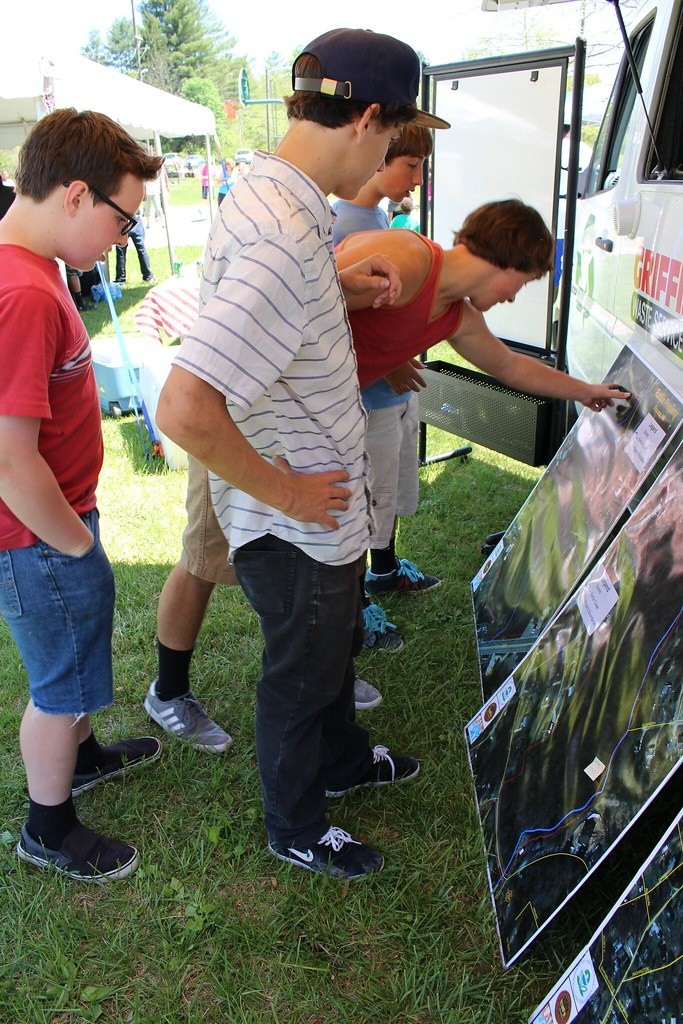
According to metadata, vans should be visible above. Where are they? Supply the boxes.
[563,0,683,421]
[234,148,253,165]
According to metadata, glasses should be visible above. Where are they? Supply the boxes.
[63,178,139,237]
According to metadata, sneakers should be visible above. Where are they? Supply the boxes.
[346,671,383,711]
[323,745,420,799]
[142,678,233,755]
[358,590,405,654]
[363,557,442,597]
[268,822,386,882]
[15,820,141,885]
[66,736,163,799]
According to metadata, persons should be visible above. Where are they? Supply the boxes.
[142,190,634,754]
[387,193,413,223]
[65,263,97,311]
[198,156,250,207]
[389,196,420,234]
[0,169,17,221]
[0,108,166,880]
[141,150,170,229]
[112,209,157,283]
[321,123,443,654]
[152,21,452,882]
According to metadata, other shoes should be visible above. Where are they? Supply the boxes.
[76,303,98,312]
[111,279,126,285]
[141,271,154,284]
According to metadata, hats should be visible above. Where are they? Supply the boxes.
[289,27,451,130]
[399,197,414,215]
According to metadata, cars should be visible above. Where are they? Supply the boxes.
[162,152,207,179]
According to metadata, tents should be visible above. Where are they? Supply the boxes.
[2,35,217,289]
